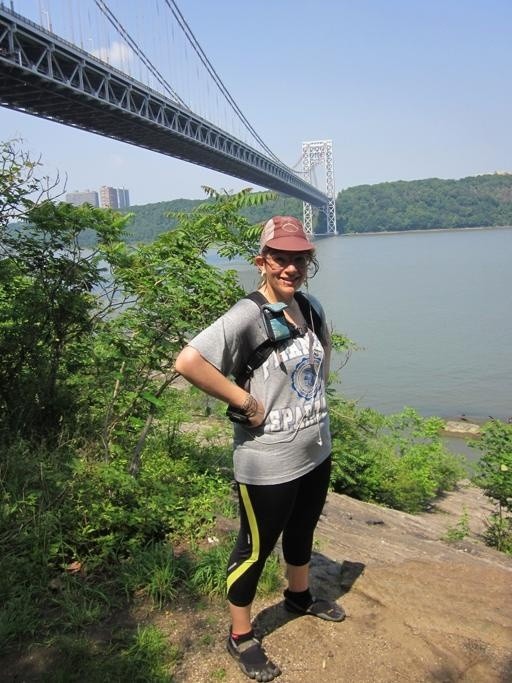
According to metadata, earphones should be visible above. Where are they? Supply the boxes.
[316,437,325,448]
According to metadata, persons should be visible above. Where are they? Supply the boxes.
[172,214,347,683]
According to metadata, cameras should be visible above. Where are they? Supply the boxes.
[225,404,248,427]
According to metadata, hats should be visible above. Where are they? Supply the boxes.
[258,216,316,254]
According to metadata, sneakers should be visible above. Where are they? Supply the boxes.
[284,592,345,622]
[227,635,280,682]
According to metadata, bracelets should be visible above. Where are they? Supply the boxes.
[240,393,258,418]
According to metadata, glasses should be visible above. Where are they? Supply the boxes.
[262,254,312,270]
